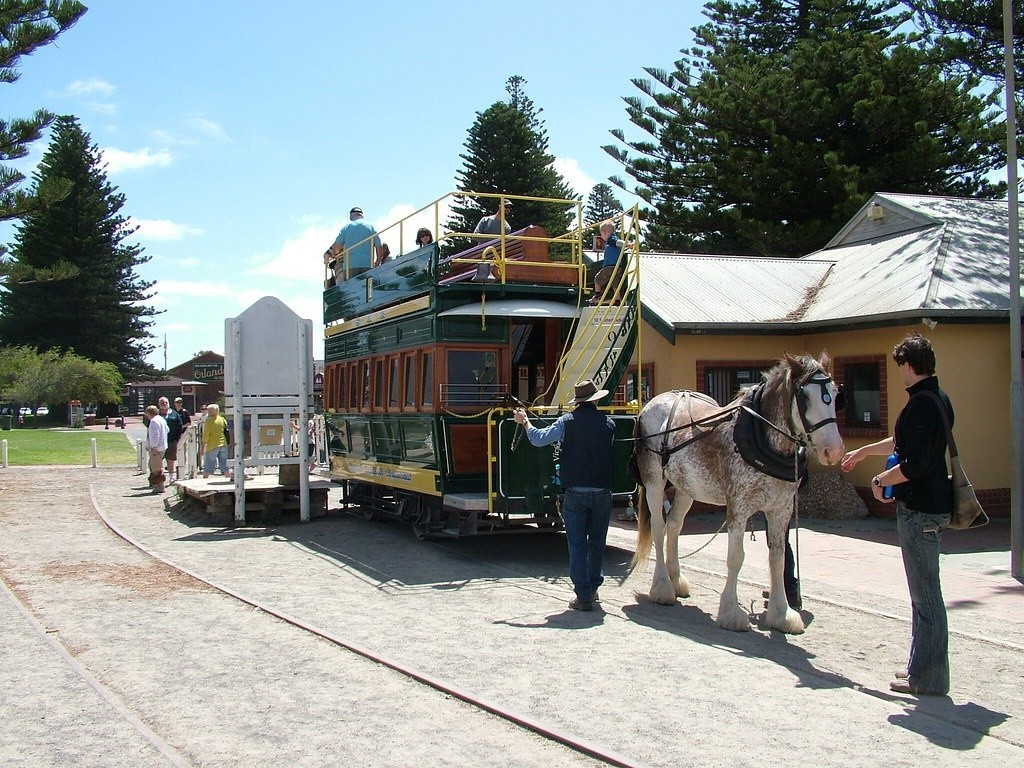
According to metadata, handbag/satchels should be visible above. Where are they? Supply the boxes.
[223,426,231,446]
[947,433,990,531]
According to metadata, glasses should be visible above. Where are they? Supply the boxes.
[202,408,206,410]
[420,233,431,239]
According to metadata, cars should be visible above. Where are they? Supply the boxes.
[20,408,32,415]
[2,408,13,415]
[37,407,48,415]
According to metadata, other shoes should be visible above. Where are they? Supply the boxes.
[586,292,604,305]
[890,667,922,695]
[570,588,600,610]
[146,482,163,495]
[612,300,621,306]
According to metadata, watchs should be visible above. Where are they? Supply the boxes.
[874,475,882,487]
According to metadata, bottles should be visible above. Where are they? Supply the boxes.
[881,448,899,498]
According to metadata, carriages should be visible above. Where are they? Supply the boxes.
[324,191,846,634]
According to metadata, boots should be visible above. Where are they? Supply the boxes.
[762,575,803,609]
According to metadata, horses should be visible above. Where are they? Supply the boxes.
[633,347,845,633]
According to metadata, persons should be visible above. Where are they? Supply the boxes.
[586,221,639,305]
[290,419,317,471]
[324,206,394,284]
[145,396,191,494]
[841,335,955,696]
[514,379,616,610]
[472,198,513,245]
[415,227,433,248]
[197,403,253,481]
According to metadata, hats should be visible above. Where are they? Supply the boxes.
[349,208,364,215]
[567,380,611,406]
[499,200,513,207]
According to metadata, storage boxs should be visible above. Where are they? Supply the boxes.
[279,464,301,486]
[260,425,282,444]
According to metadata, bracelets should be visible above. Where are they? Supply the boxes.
[525,421,528,425]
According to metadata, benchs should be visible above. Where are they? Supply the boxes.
[438,226,579,285]
